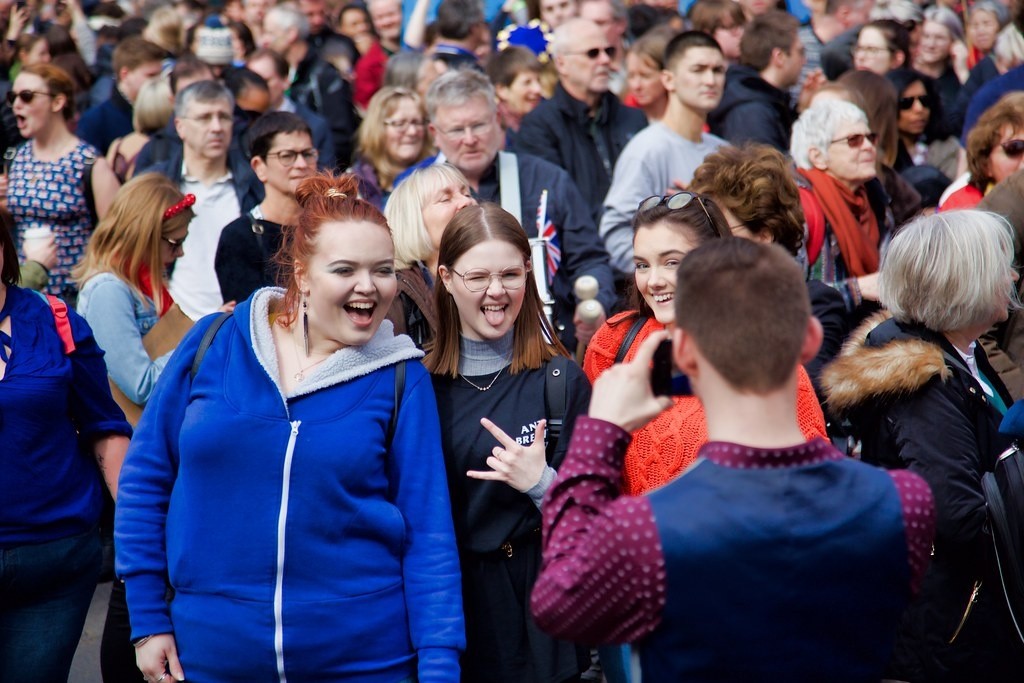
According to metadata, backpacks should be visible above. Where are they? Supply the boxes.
[982,441,1024,643]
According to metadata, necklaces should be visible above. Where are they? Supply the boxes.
[458,352,512,391]
[292,332,325,380]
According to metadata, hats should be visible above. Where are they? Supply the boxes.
[191,15,234,65]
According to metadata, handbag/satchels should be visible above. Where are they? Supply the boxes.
[100,578,174,683]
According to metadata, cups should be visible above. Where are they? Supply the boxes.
[23,227,52,254]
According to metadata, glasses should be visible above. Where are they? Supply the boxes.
[438,122,494,139]
[895,94,933,109]
[850,45,895,56]
[566,46,615,58]
[385,119,430,131]
[1000,139,1024,156]
[161,233,184,253]
[451,268,532,292]
[830,133,879,147]
[6,90,55,108]
[639,192,719,237]
[264,147,319,165]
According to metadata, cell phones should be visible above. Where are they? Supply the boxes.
[649,338,695,396]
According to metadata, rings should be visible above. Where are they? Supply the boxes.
[144,676,148,680]
[497,450,504,458]
[158,673,166,681]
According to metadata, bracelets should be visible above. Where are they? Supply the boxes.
[133,635,153,648]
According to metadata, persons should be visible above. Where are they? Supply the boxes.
[0,0,1024,683]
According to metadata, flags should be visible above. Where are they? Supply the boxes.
[535,187,561,287]
